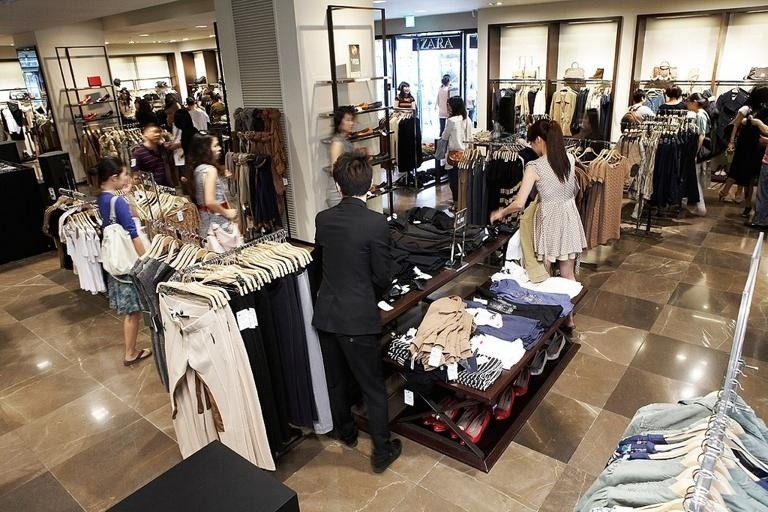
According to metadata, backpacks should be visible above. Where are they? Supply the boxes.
[621,106,641,132]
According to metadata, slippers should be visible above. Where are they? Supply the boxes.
[124,350,152,366]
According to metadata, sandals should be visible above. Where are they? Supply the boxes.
[720,192,743,203]
[449,203,458,212]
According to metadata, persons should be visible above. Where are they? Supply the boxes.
[490,119,588,330]
[394,82,416,108]
[97,156,153,366]
[312,153,401,474]
[441,95,474,211]
[326,106,367,209]
[118,86,227,185]
[628,88,655,130]
[718,86,768,228]
[684,92,712,215]
[437,73,477,133]
[183,133,243,252]
[655,85,688,116]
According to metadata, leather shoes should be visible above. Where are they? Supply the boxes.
[369,152,388,160]
[367,182,390,198]
[688,207,707,216]
[350,127,379,139]
[357,101,381,109]
[743,207,767,229]
[424,398,493,445]
[495,367,531,419]
[346,438,402,472]
[79,94,113,120]
[530,333,566,375]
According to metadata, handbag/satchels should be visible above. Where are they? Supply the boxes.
[102,196,152,275]
[207,223,244,254]
[747,67,768,79]
[653,62,676,79]
[697,113,722,164]
[565,63,584,77]
[448,151,463,166]
[512,57,538,80]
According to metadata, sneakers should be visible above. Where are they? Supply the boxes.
[711,165,727,182]
